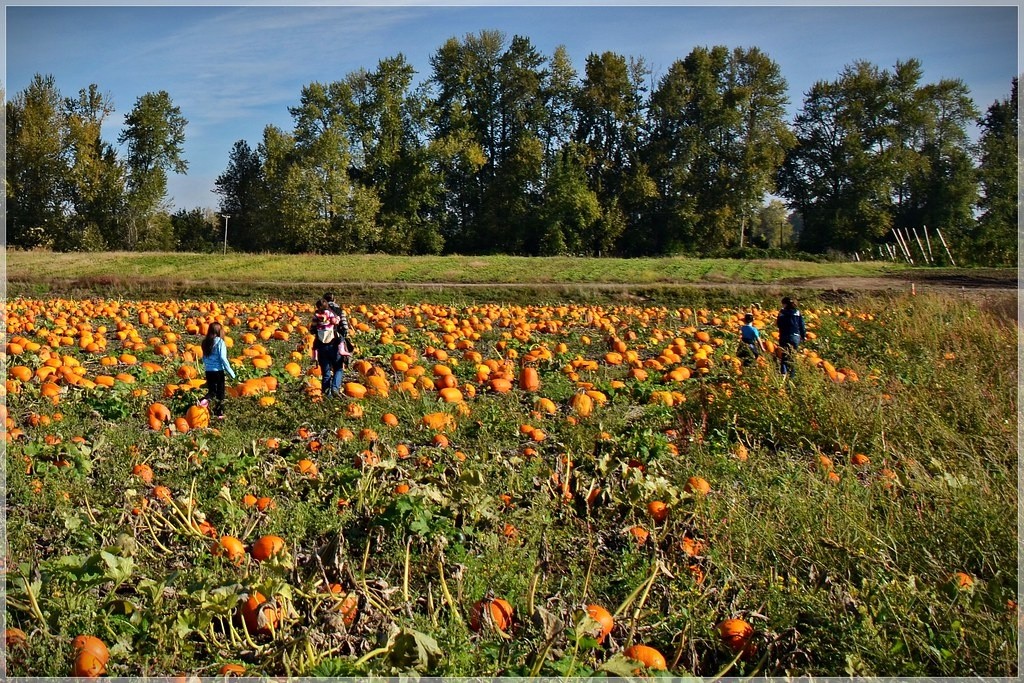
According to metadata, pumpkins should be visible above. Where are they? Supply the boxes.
[0,298,973,682]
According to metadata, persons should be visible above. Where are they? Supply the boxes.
[199,321,236,419]
[309,291,354,400]
[776,297,807,378]
[739,313,766,367]
